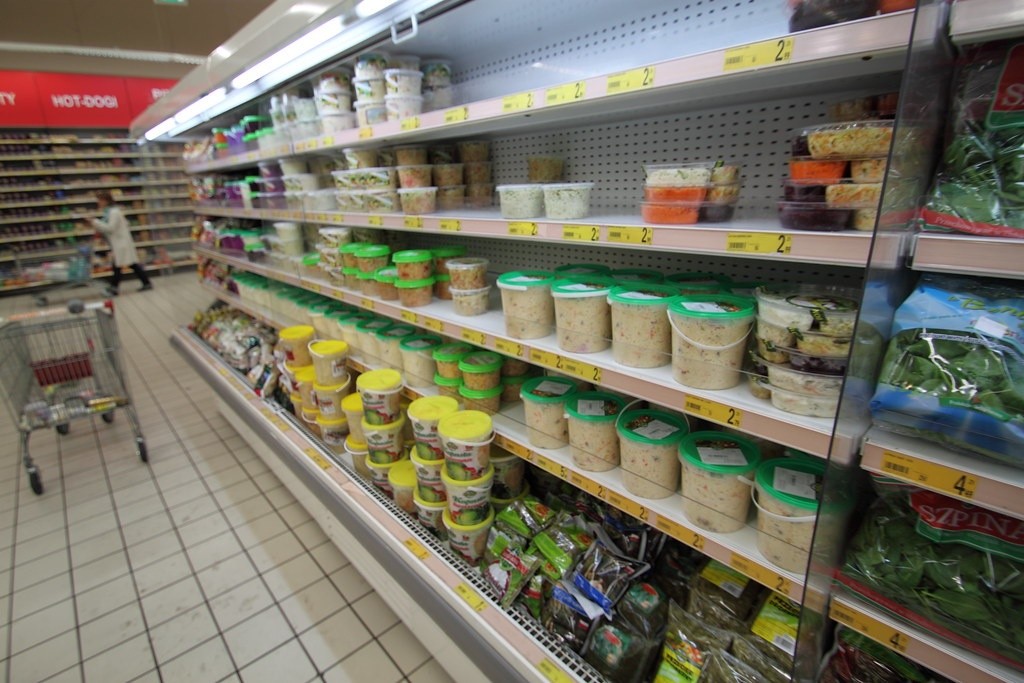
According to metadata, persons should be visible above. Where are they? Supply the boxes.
[84,193,153,296]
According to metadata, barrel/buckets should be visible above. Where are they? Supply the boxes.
[209,49,850,575]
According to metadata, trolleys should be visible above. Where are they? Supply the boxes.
[1,300,149,496]
[10,231,109,306]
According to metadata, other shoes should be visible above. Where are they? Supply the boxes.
[136,283,152,293]
[104,285,120,296]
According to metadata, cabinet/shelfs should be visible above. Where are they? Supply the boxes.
[0,138,198,293]
[182,0,1024,683]
